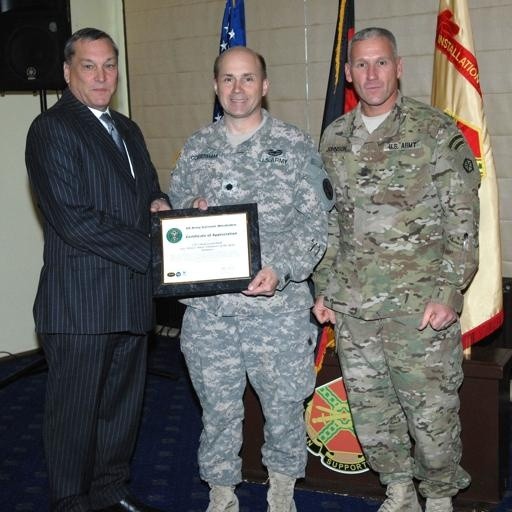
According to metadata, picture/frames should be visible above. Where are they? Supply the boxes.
[151,203,262,299]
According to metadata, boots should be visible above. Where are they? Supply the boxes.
[265,476,299,511]
[425,496,453,512]
[377,478,423,512]
[205,482,239,512]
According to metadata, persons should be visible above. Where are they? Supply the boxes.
[23,27,177,511]
[308,25,483,511]
[166,44,340,512]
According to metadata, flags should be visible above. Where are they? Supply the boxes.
[211,1,248,123]
[300,1,357,380]
[428,1,509,361]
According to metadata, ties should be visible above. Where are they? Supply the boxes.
[98,112,131,173]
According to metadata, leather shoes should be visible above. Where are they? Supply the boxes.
[99,494,161,512]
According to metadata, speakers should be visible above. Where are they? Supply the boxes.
[0,0,72,91]
